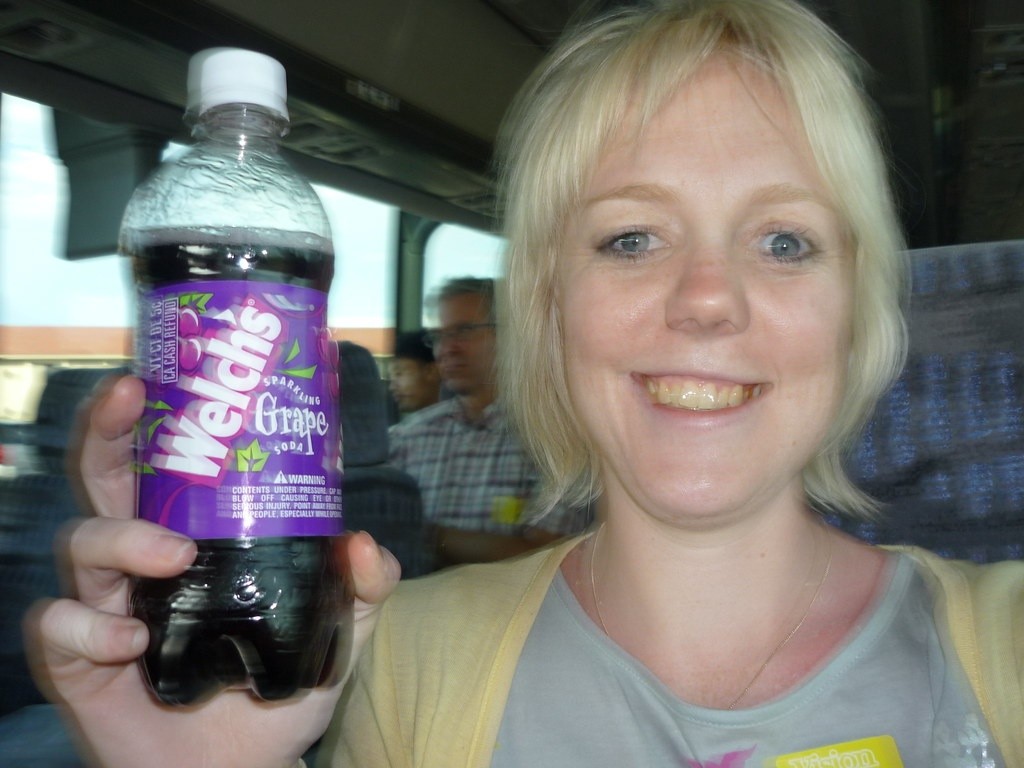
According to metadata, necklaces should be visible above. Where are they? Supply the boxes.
[589,518,836,711]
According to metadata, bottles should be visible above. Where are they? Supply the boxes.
[115,46,346,711]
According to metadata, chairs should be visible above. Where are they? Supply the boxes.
[0,240,1024,768]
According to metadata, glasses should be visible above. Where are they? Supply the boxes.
[422,323,495,348]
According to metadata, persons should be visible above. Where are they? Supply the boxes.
[20,0,1024,768]
[387,276,589,563]
[388,327,442,415]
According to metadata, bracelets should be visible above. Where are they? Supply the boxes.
[428,525,447,556]
